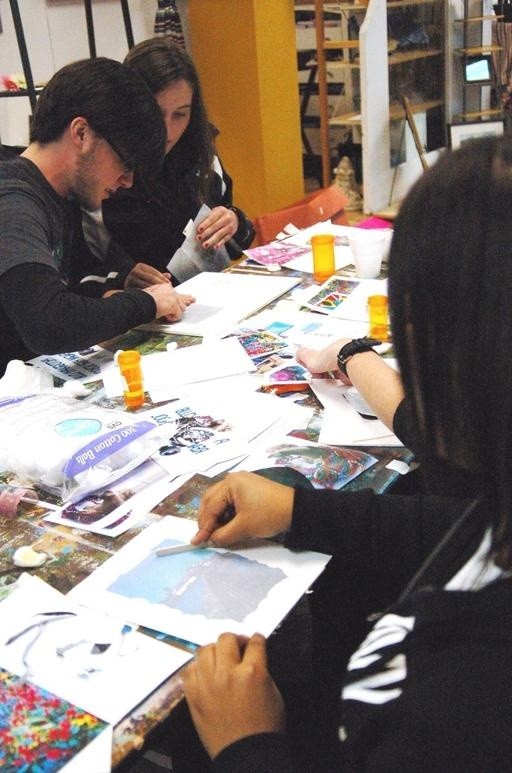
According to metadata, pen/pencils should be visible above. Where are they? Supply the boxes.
[19,497,64,512]
[155,541,206,557]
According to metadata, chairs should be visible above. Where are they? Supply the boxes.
[255,187,348,247]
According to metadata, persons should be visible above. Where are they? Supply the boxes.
[1,58,185,378]
[297,339,429,456]
[184,132,512,773]
[100,38,255,285]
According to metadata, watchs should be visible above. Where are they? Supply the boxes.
[338,336,382,377]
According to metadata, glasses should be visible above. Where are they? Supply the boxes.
[102,133,138,172]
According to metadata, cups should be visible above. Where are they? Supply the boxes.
[116,350,145,412]
[311,233,337,281]
[367,296,390,344]
[349,230,386,278]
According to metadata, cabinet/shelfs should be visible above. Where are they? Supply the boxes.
[312,1,504,194]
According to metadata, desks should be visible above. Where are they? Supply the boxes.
[1,213,412,773]
